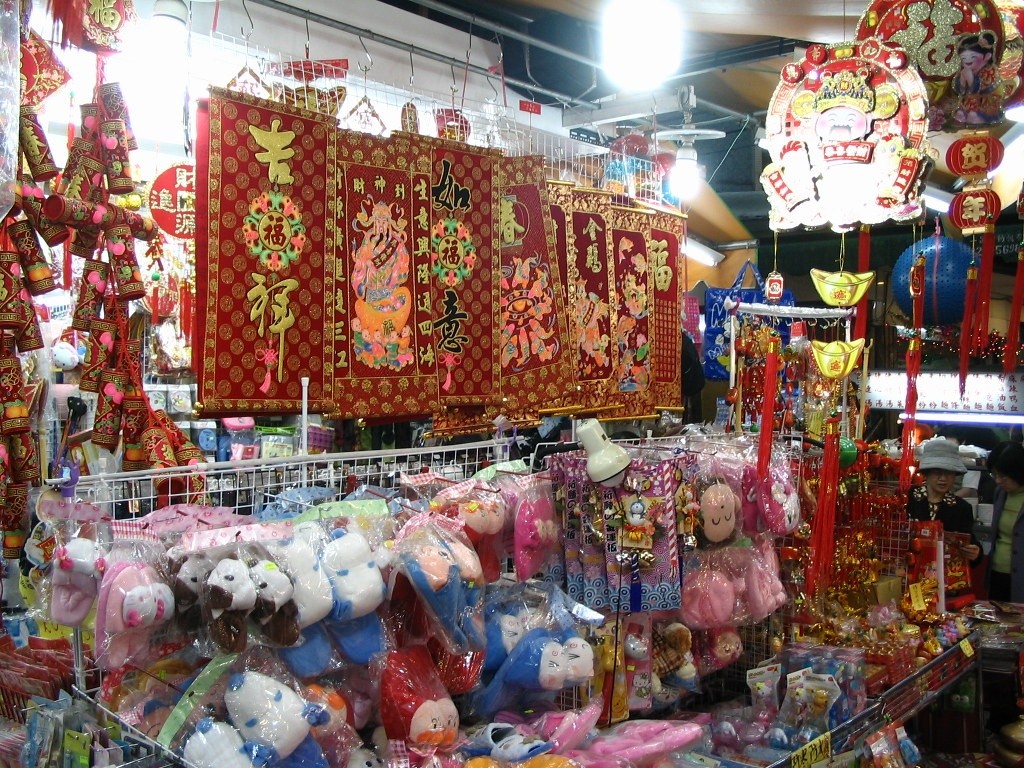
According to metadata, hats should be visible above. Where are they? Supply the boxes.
[918,440,968,475]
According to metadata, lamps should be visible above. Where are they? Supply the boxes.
[534,420,633,483]
[152,0,188,36]
[651,110,727,198]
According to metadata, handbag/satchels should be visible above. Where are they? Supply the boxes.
[703,260,792,383]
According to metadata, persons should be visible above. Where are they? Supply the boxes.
[979,441,1024,604]
[905,439,984,601]
[947,432,981,500]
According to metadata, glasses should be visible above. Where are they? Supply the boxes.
[990,470,1009,481]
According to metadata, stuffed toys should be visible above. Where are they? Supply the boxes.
[650,456,801,704]
[53,477,702,768]
